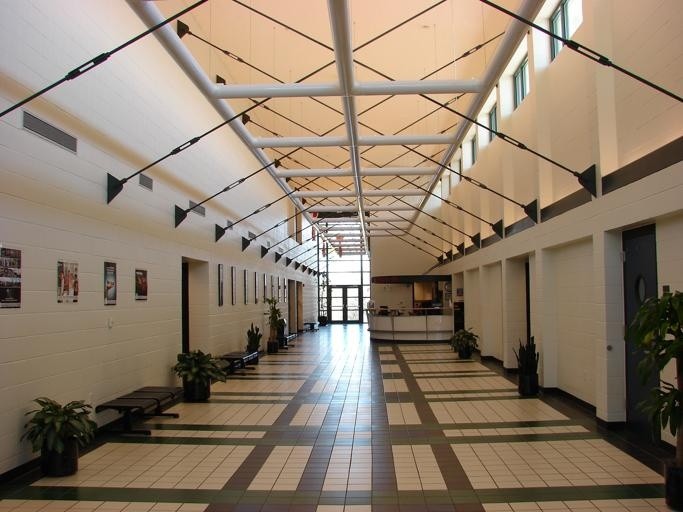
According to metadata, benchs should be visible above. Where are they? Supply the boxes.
[304,323,319,332]
[222,352,258,375]
[96,386,181,435]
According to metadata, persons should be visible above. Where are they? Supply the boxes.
[57,268,78,302]
[141,274,147,296]
[0,248,20,302]
[136,274,142,295]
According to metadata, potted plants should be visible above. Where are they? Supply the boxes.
[172,350,230,401]
[448,328,480,359]
[20,397,98,477]
[512,336,539,397]
[246,322,262,365]
[264,297,286,353]
[624,290,683,512]
